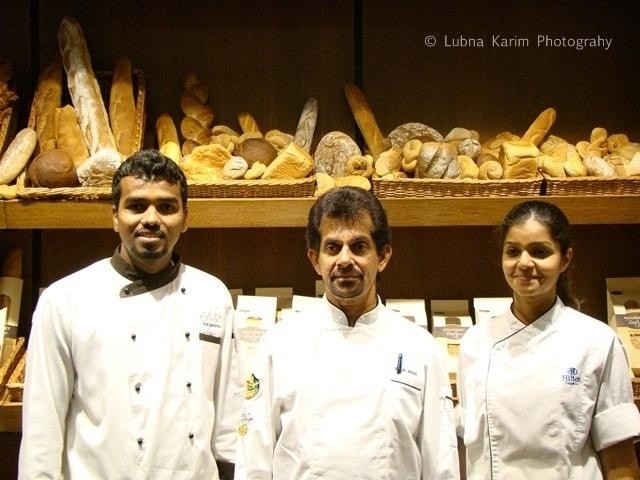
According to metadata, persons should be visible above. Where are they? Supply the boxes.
[17,148,244,480]
[457,201,640,479]
[234,182,462,479]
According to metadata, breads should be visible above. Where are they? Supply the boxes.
[1,249,28,406]
[1,18,639,198]
